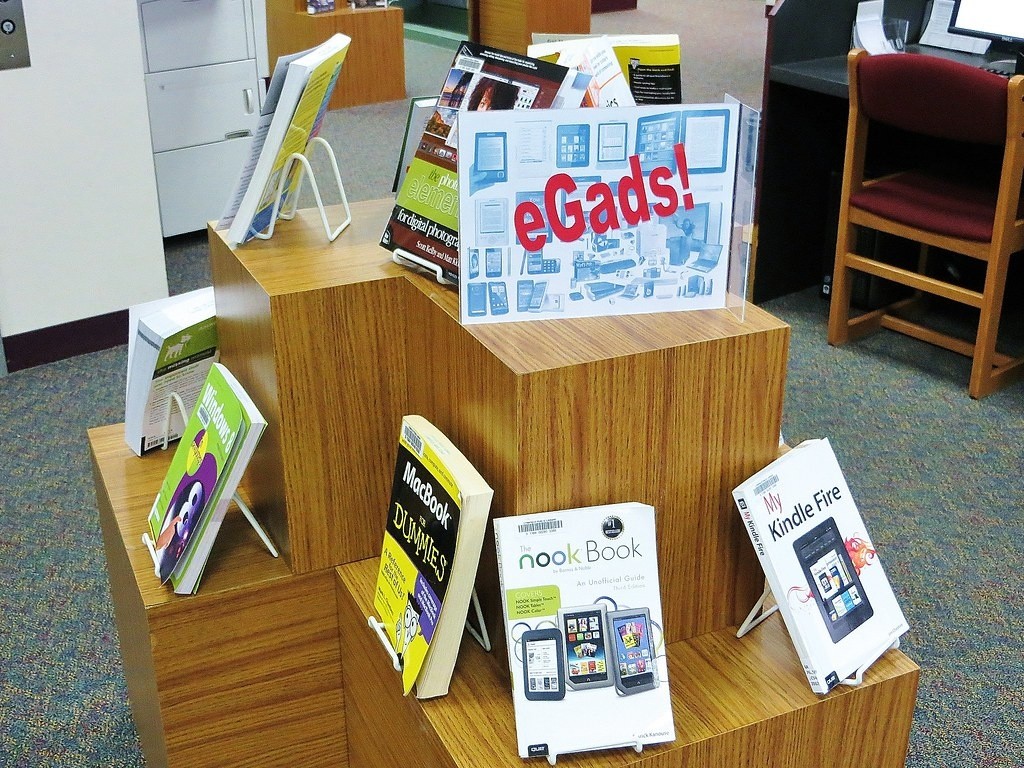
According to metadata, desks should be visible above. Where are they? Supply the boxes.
[748,0,1023,333]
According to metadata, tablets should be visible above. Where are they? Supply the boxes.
[521,604,660,700]
[792,518,875,644]
[468,109,730,316]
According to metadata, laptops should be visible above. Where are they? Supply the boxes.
[687,246,723,274]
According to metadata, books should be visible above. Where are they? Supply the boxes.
[734,437,910,695]
[124,285,217,457]
[493,501,676,758]
[215,33,351,244]
[147,363,268,595]
[373,414,494,699]
[379,33,682,287]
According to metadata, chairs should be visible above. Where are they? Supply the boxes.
[828,48,1024,399]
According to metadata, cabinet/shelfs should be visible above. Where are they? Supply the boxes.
[137,0,270,240]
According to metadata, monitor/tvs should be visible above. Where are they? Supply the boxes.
[947,0,1024,54]
